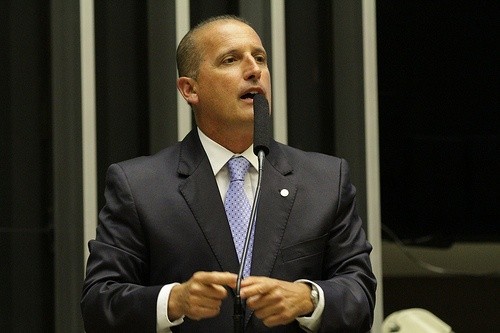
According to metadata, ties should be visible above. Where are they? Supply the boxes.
[223,158,256,279]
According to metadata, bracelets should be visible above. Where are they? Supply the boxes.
[299,283,321,320]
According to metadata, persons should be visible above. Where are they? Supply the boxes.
[84,14,377,332]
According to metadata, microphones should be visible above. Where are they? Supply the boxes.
[253,94,270,154]
